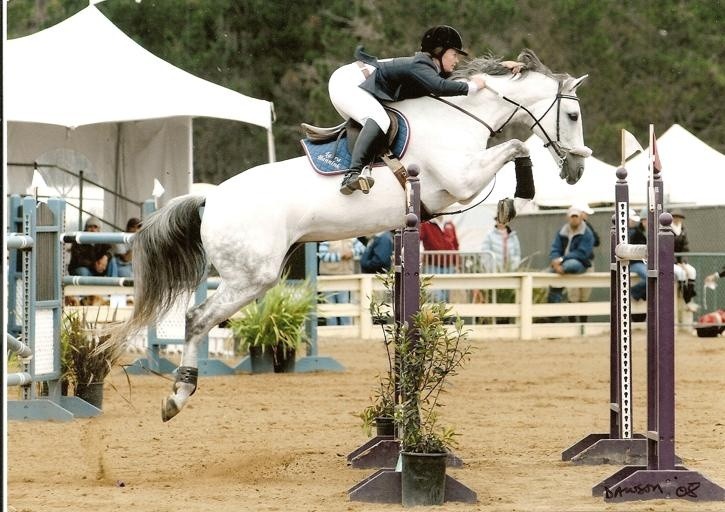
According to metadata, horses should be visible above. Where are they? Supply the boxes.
[76,47,589,423]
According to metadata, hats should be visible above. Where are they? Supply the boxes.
[85,216,101,228]
[128,217,143,228]
[567,203,596,219]
[626,208,689,222]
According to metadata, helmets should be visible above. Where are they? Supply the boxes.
[420,26,469,58]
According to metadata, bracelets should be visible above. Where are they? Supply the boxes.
[558,257,564,263]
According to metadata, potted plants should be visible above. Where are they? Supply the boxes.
[224,263,341,376]
[14,306,174,410]
[348,252,477,506]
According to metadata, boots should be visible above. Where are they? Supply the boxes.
[683,279,700,313]
[340,120,388,195]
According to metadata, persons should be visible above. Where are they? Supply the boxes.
[619,205,725,316]
[113,217,144,278]
[315,237,367,325]
[327,24,524,196]
[417,214,461,323]
[360,229,393,325]
[479,218,521,274]
[67,216,118,278]
[567,203,602,322]
[547,206,596,322]
